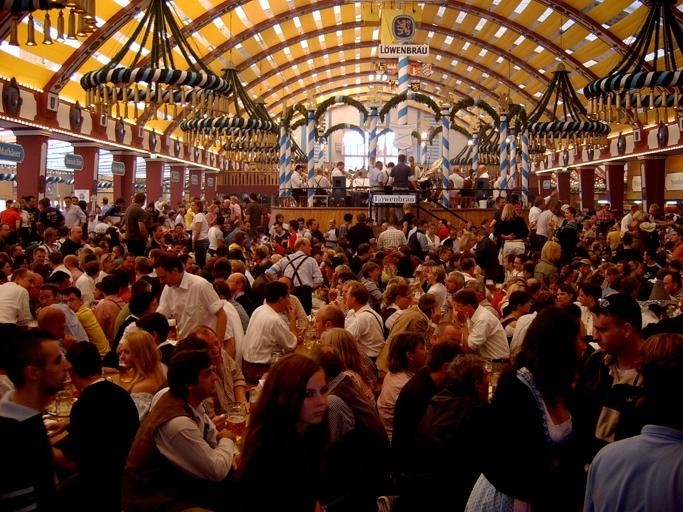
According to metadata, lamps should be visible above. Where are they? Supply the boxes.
[447,109,546,172]
[79,1,229,120]
[0,1,98,48]
[583,2,682,129]
[516,65,611,154]
[418,157,450,185]
[180,64,277,148]
[216,98,306,175]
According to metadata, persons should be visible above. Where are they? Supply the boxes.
[289,151,489,208]
[1,183,683,512]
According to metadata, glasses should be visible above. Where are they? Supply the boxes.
[597,297,614,312]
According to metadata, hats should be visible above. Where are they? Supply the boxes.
[578,258,591,265]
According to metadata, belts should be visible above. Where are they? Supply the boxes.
[492,358,511,363]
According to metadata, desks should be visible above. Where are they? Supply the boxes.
[27,264,456,469]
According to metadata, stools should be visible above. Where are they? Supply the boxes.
[274,188,493,211]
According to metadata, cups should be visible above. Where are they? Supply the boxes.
[483,359,501,399]
[61,374,73,390]
[166,318,177,338]
[118,356,134,384]
[296,314,322,354]
[486,279,494,298]
[54,390,72,424]
[224,399,247,443]
